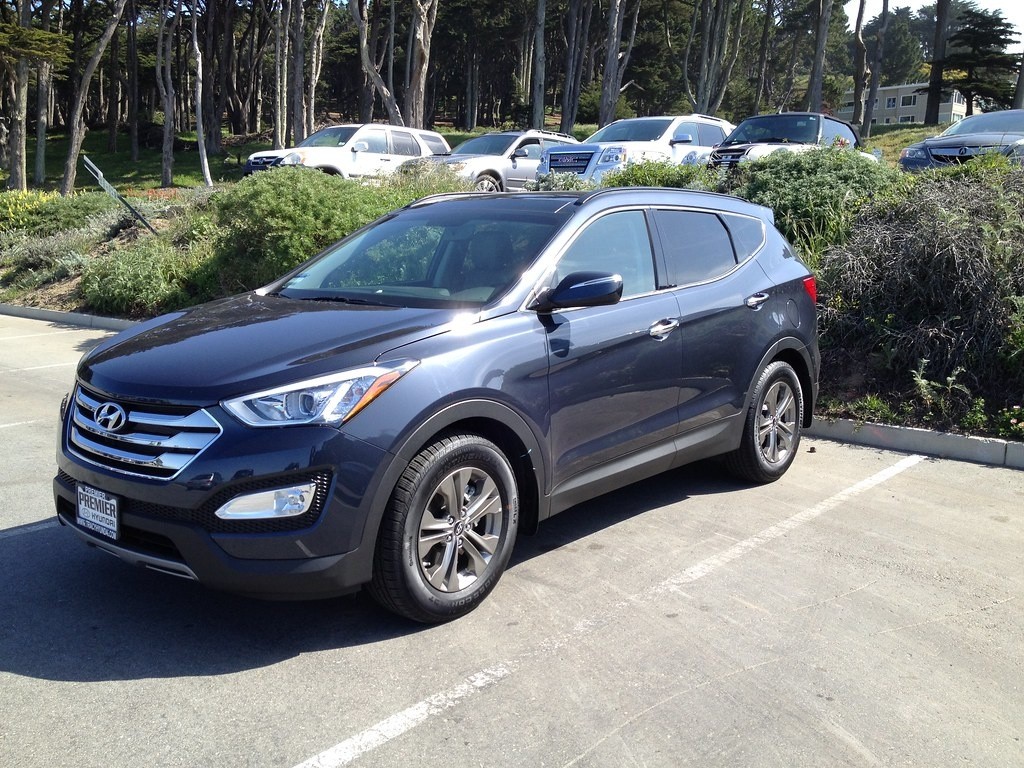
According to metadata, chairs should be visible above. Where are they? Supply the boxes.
[462,231,513,289]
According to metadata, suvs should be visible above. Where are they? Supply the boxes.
[389,127,582,190]
[242,123,453,187]
[706,108,879,182]
[534,112,747,191]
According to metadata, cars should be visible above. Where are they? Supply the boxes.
[50,185,822,626]
[895,108,1024,176]
